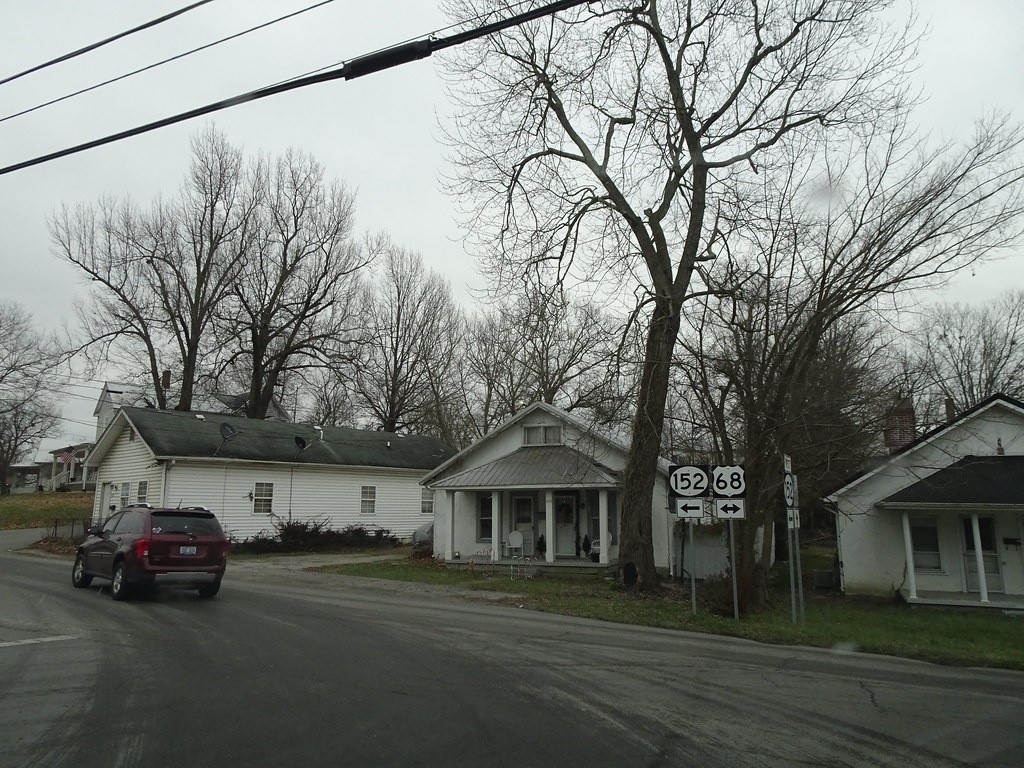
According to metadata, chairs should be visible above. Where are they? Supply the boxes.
[505,531,523,559]
[592,532,613,553]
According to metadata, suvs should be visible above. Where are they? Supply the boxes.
[72,503,231,602]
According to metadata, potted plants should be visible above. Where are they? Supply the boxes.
[582,534,591,559]
[536,533,546,558]
